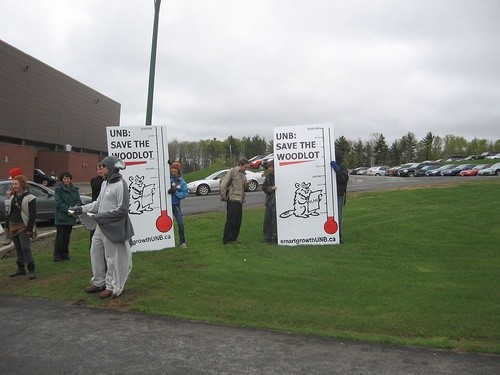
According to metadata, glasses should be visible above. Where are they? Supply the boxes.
[102,163,105,167]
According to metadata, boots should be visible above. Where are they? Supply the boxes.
[27,262,36,279]
[9,261,26,277]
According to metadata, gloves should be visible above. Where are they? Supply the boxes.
[330,161,341,172]
[68,206,83,216]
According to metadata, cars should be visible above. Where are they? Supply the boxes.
[186,167,266,196]
[349,150,500,177]
[248,152,274,169]
[0,179,92,225]
[34,167,58,186]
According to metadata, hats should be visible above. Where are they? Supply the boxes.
[171,162,181,170]
[9,168,22,178]
[101,155,126,181]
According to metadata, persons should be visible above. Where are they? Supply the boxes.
[69,154,135,298]
[0,150,348,280]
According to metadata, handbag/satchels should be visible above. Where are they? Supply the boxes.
[21,211,38,239]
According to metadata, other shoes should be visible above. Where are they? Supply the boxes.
[85,286,104,291]
[99,289,113,298]
[180,243,187,248]
